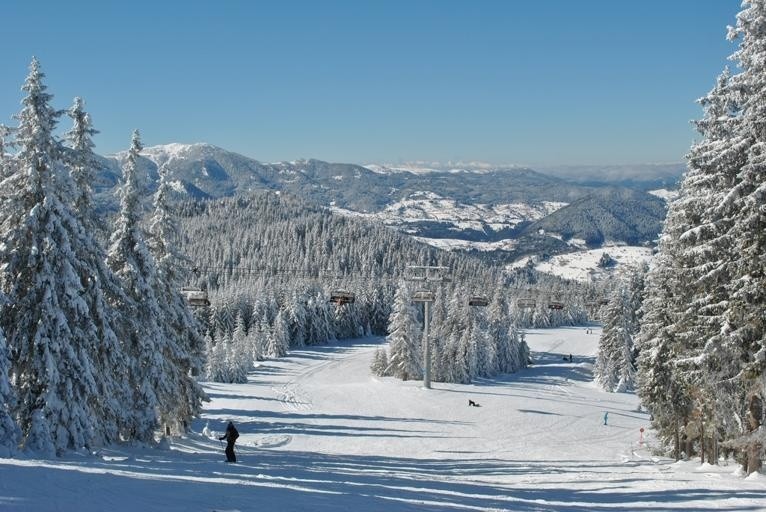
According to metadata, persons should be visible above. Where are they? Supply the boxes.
[605,411,608,426]
[219,421,239,462]
[570,354,572,362]
[469,399,480,407]
